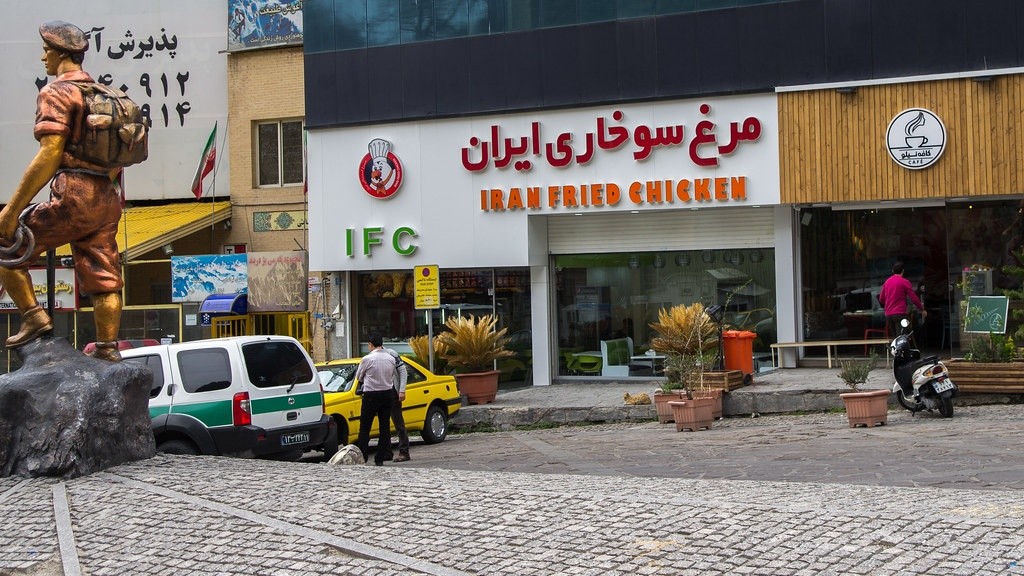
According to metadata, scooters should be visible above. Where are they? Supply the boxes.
[885,318,959,418]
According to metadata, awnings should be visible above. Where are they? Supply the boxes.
[37,200,232,263]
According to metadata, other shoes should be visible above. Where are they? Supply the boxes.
[393,453,411,461]
[375,459,383,466]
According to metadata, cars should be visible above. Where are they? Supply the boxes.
[313,355,461,446]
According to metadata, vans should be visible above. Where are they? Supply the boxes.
[81,335,335,461]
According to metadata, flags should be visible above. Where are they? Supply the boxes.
[192,121,218,199]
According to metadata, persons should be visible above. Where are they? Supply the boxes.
[879,262,927,349]
[0,21,124,364]
[356,332,410,466]
